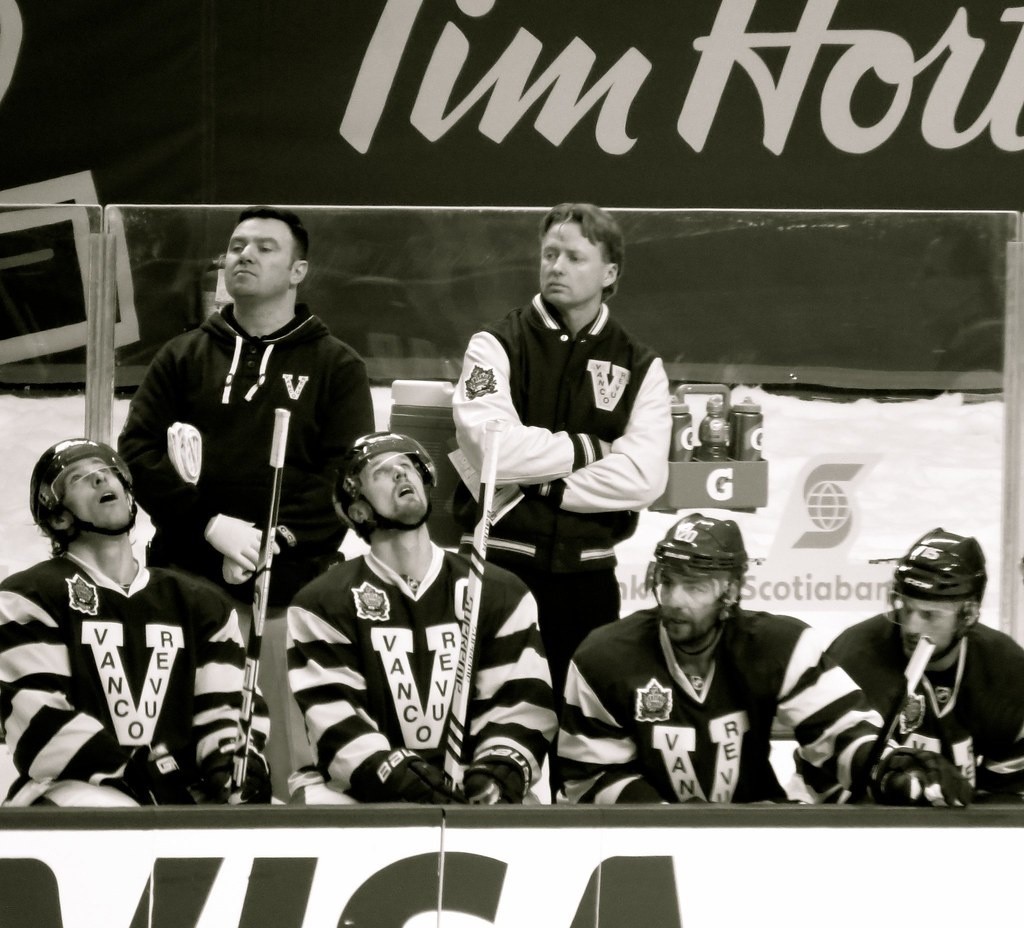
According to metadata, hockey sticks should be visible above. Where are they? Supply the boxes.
[849,640,936,803]
[226,407,294,807]
[442,422,504,797]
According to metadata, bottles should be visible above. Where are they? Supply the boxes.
[669,396,694,461]
[699,397,731,462]
[730,397,765,462]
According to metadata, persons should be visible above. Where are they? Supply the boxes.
[792,524,1024,807]
[556,512,982,809]
[449,199,675,706]
[280,430,554,805]
[114,204,378,811]
[0,439,271,807]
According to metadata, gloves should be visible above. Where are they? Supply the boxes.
[123,743,196,804]
[214,735,272,804]
[365,747,454,804]
[204,514,280,572]
[871,746,971,808]
[461,746,532,805]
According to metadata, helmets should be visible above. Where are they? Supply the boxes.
[892,527,988,601]
[654,512,748,571]
[331,431,437,540]
[30,437,133,545]
